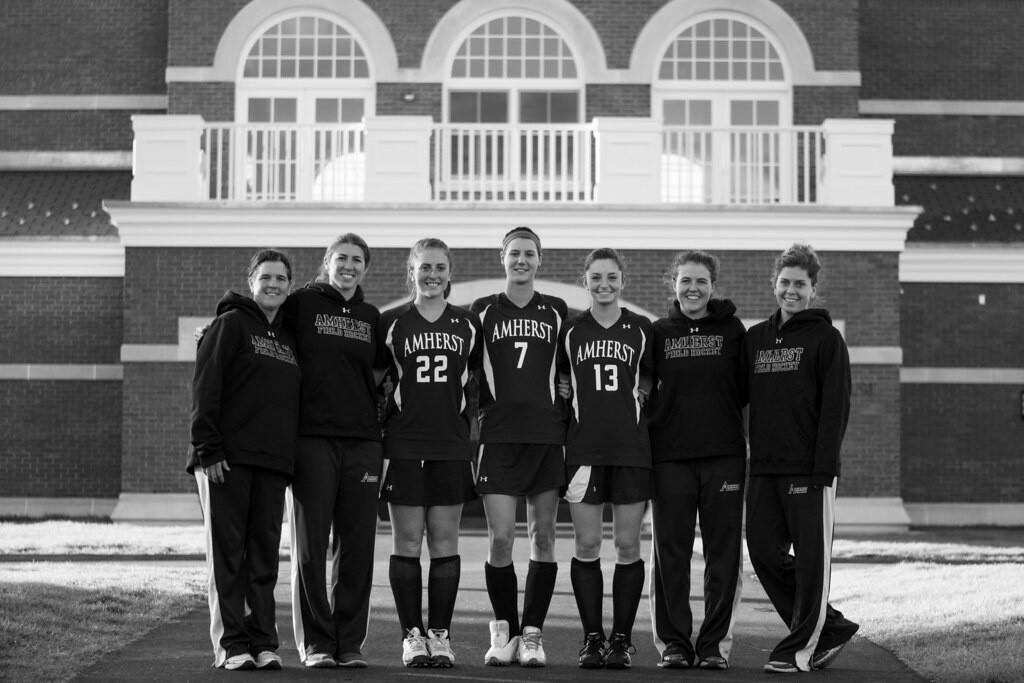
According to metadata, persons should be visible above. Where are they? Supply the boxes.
[192,232,382,667]
[378,226,651,672]
[185,250,298,670]
[745,244,858,671]
[651,251,745,670]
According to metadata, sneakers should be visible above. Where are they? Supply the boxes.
[426,628,456,668]
[254,649,284,671]
[604,636,637,668]
[518,626,547,667]
[402,627,431,668]
[483,620,519,666]
[812,638,852,670]
[222,651,257,670]
[303,651,337,669]
[699,654,728,669]
[661,650,689,669]
[338,650,368,669]
[578,634,607,669]
[763,658,799,673]
